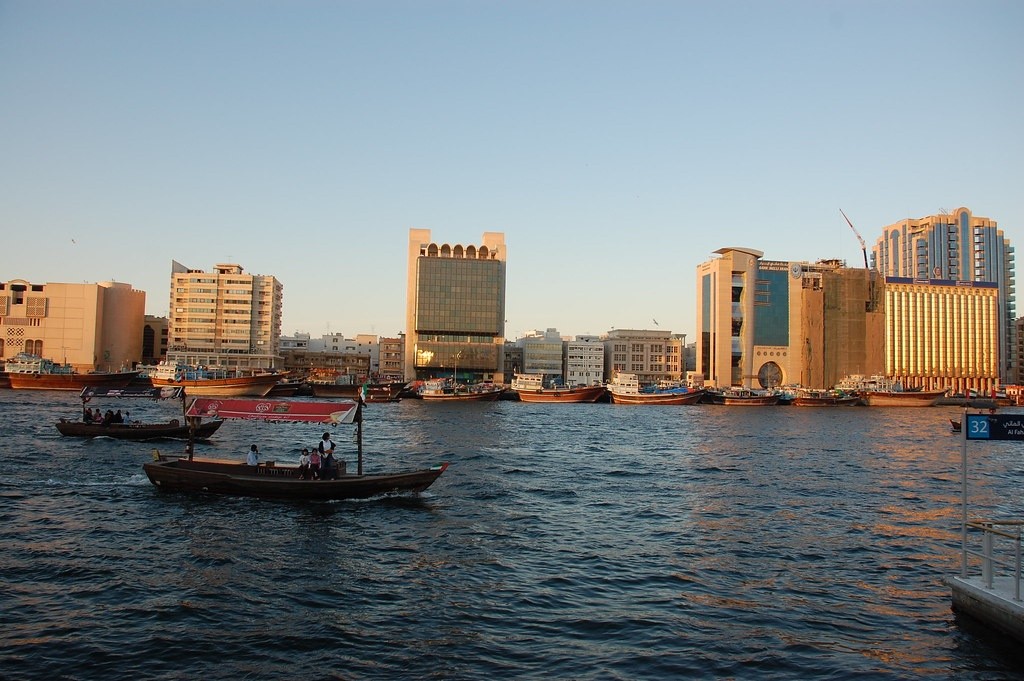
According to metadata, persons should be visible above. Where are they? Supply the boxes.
[160,387,179,400]
[247,445,263,476]
[83,408,129,428]
[299,448,320,480]
[319,432,334,480]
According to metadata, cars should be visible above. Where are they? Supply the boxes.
[969,390,976,398]
[954,393,964,398]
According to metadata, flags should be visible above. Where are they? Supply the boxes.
[360,383,367,401]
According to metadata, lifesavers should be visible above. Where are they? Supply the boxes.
[358,387,362,393]
[554,393,557,397]
[570,390,572,393]
[888,393,893,398]
[32,373,38,378]
[536,389,541,393]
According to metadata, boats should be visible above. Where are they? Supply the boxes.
[859,379,950,407]
[266,371,410,398]
[711,389,782,407]
[604,373,706,405]
[415,380,511,402]
[55,383,224,441]
[949,403,999,431]
[512,371,607,403]
[0,353,143,390]
[792,391,860,405]
[142,385,449,500]
[149,361,290,397]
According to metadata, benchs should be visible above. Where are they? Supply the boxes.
[256,462,298,478]
